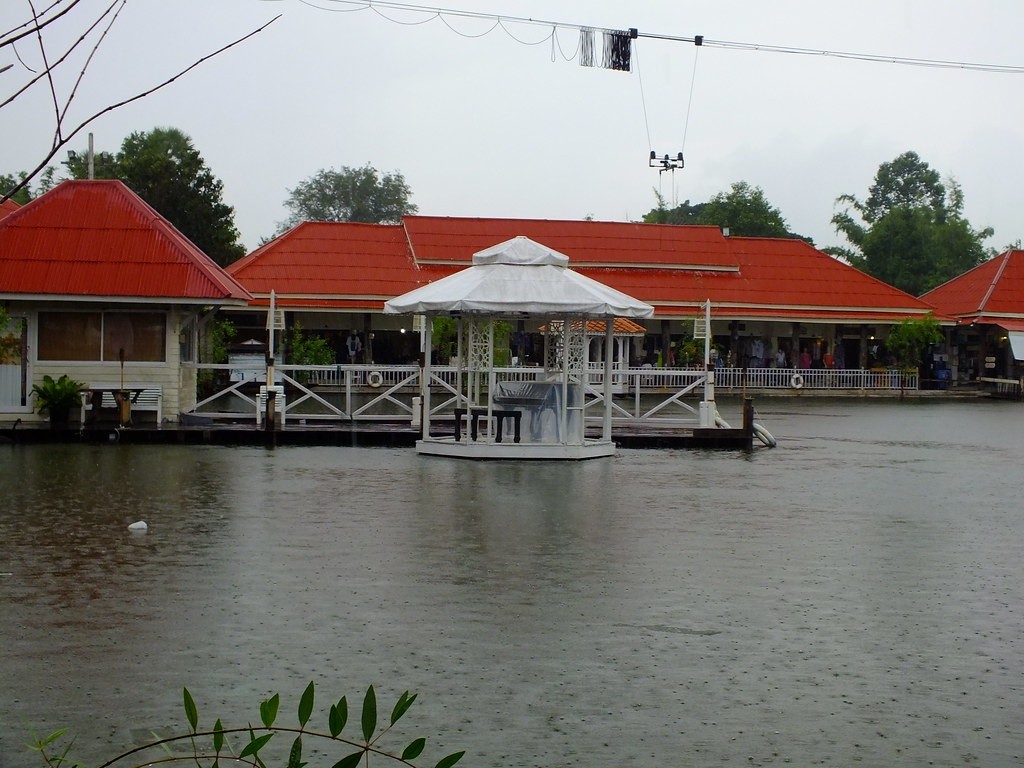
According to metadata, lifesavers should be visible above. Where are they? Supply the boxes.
[368,372,383,387]
[753,422,777,446]
[715,416,731,428]
[791,374,804,389]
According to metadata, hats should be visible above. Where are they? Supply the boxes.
[351,329,357,335]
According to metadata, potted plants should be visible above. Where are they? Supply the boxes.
[28,375,86,424]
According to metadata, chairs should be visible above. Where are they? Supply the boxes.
[256,385,286,425]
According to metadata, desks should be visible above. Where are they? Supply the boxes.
[82,389,160,426]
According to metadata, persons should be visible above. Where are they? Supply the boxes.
[322,329,1024,386]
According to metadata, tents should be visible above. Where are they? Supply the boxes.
[384,236,655,446]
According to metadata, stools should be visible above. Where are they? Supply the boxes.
[454,408,522,443]
